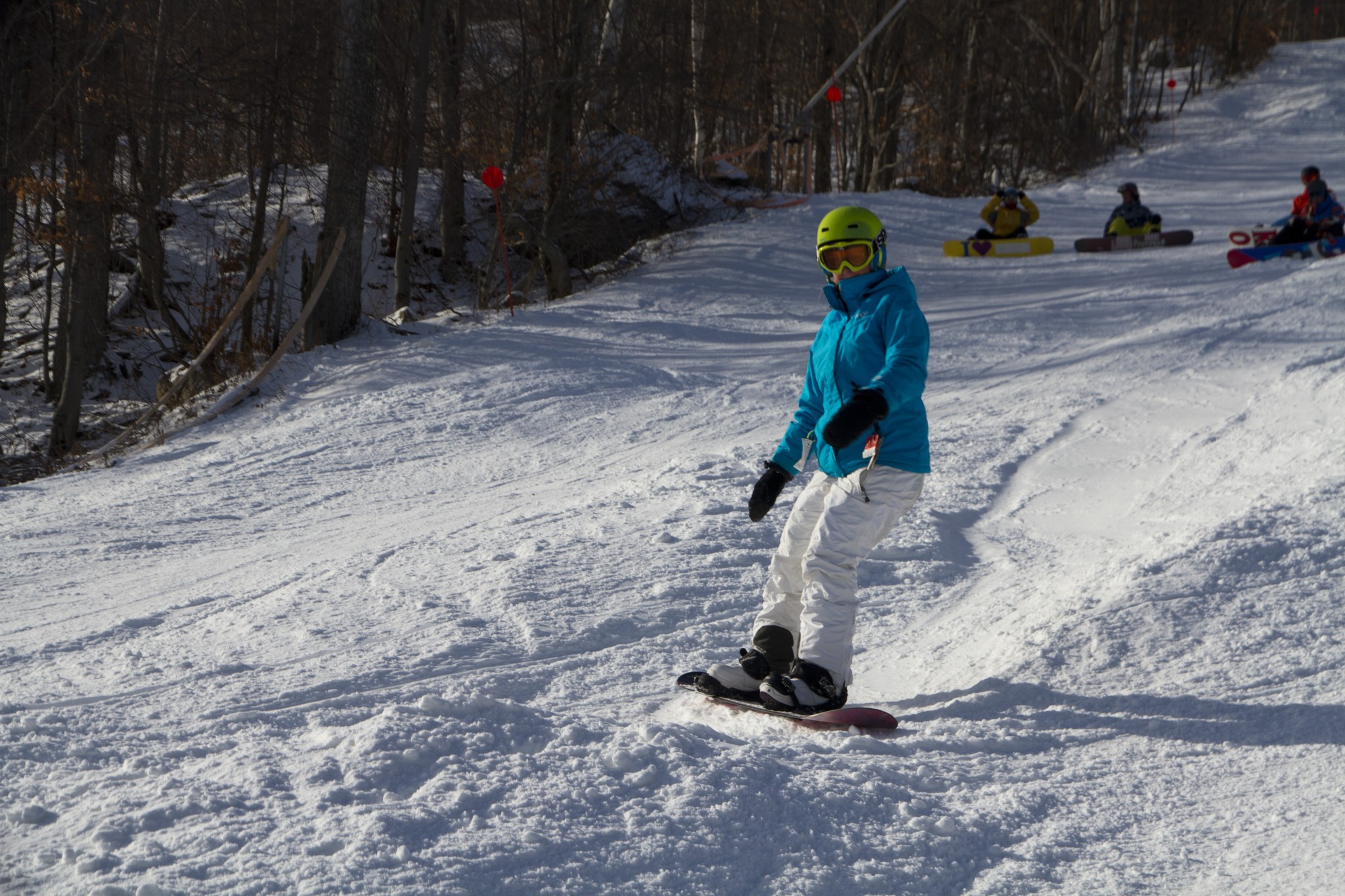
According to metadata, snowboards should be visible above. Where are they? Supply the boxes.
[1074,229,1195,253]
[676,671,898,729]
[943,237,1053,257]
[1226,237,1345,269]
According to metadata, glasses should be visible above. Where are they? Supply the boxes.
[816,241,873,275]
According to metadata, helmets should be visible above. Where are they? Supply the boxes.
[816,205,887,282]
[1308,181,1326,194]
[1118,182,1138,195]
[1300,166,1319,179]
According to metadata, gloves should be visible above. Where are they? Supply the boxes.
[997,189,1004,197]
[1018,191,1024,199]
[748,462,792,522]
[821,390,886,451]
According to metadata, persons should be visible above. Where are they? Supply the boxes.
[707,205,931,706]
[980,187,1040,239]
[1103,183,1161,237]
[1291,165,1345,243]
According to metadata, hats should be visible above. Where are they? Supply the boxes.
[1004,187,1018,199]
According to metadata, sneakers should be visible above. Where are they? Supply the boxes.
[707,660,762,692]
[759,677,828,708]
